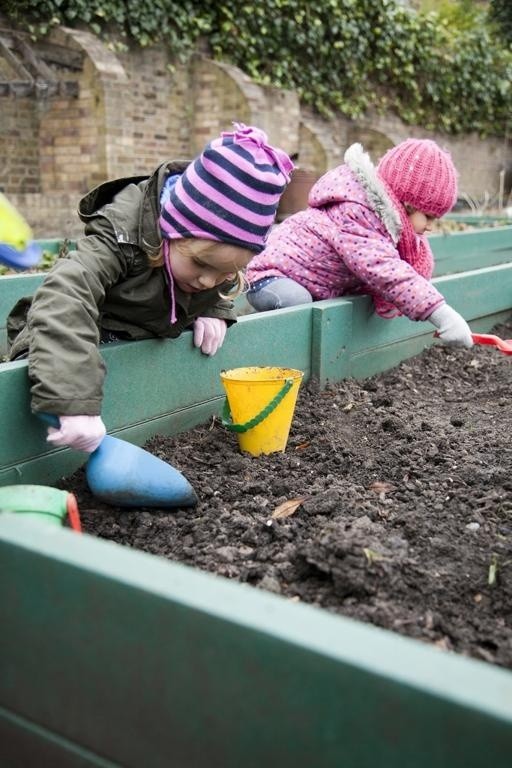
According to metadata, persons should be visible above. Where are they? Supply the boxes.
[3,117,299,455]
[244,137,475,351]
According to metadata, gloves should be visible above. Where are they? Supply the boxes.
[192,316,227,356]
[46,414,106,453]
[427,303,474,349]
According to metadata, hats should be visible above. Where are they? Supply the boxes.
[160,122,295,253]
[376,138,458,218]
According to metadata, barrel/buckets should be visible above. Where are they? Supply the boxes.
[214,365,304,458]
[0,483,81,533]
